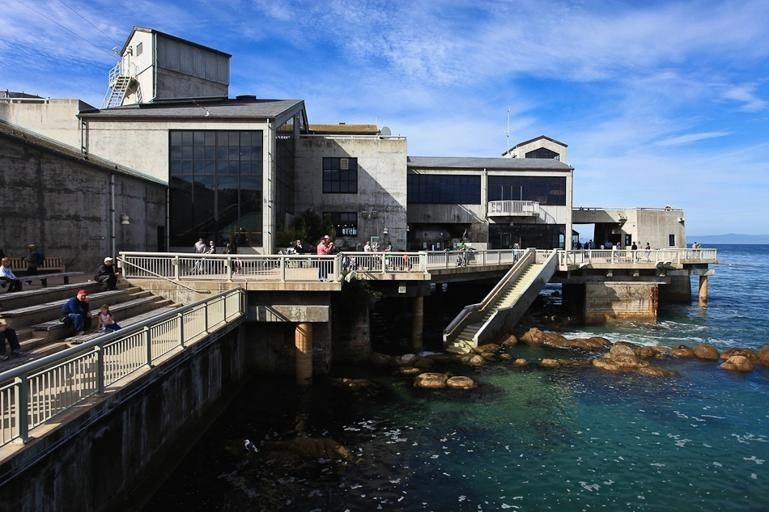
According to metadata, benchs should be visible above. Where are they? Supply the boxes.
[0,253,175,373]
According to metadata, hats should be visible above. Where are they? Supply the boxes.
[104,257,113,262]
[79,290,87,295]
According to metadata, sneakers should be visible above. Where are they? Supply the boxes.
[0,354,9,360]
[13,350,28,357]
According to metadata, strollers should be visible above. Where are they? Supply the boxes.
[516,251,524,261]
[391,249,412,271]
[190,254,211,274]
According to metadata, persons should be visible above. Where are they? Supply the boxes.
[59,290,92,335]
[0,257,22,293]
[692,242,699,249]
[94,257,120,292]
[571,240,652,263]
[364,241,371,253]
[316,235,334,282]
[384,241,392,252]
[0,314,28,361]
[97,304,122,334]
[193,238,237,254]
[24,243,39,276]
